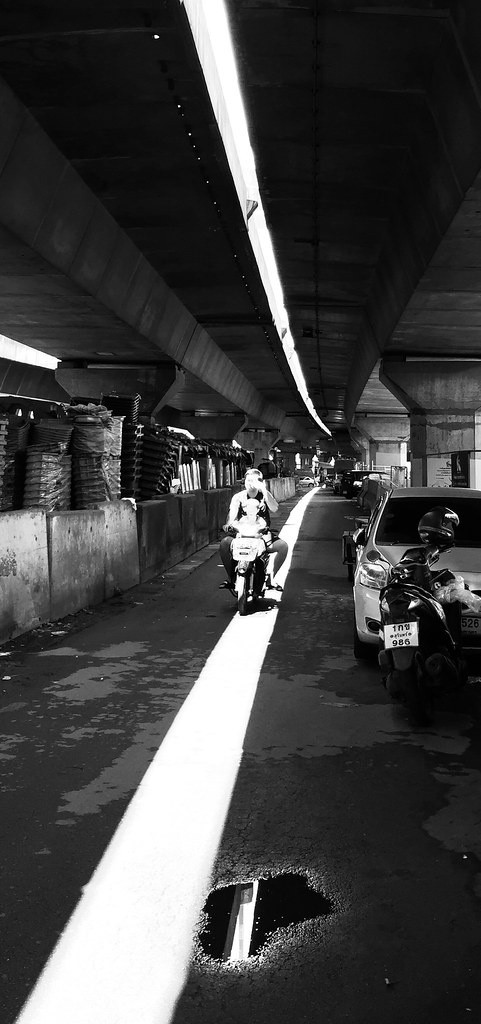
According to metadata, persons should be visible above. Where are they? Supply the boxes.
[218,468,289,592]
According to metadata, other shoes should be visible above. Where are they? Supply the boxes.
[218,580,235,590]
[266,577,284,592]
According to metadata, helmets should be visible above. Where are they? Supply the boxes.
[418,511,454,547]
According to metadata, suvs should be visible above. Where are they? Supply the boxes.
[350,487,481,667]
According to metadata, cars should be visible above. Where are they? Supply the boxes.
[298,476,319,487]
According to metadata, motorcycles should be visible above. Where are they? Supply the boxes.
[363,537,455,699]
[224,526,279,613]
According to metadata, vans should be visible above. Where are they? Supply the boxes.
[332,472,343,496]
[342,470,380,499]
[325,474,334,488]
